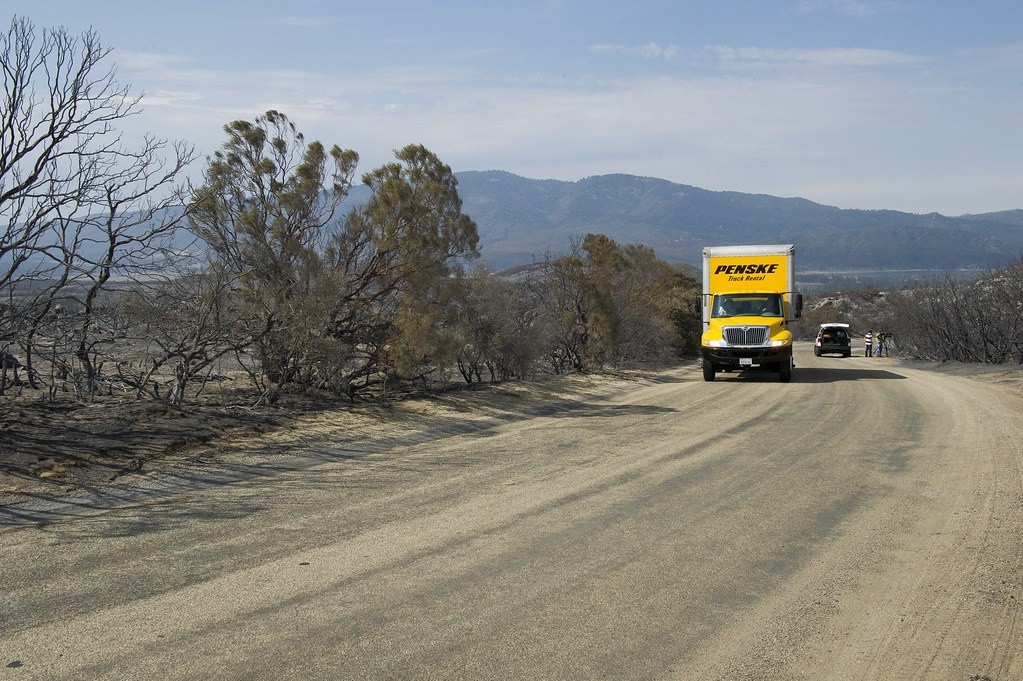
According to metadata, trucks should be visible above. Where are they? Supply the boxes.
[701,244,804,380]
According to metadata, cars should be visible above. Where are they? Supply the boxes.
[815,321,854,357]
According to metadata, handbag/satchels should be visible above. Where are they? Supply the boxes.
[876,348,880,357]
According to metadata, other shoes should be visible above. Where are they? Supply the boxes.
[865,356,868,357]
[869,355,873,357]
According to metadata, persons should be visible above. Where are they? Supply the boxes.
[717,298,737,316]
[876,330,890,357]
[864,329,873,357]
[762,297,784,317]
